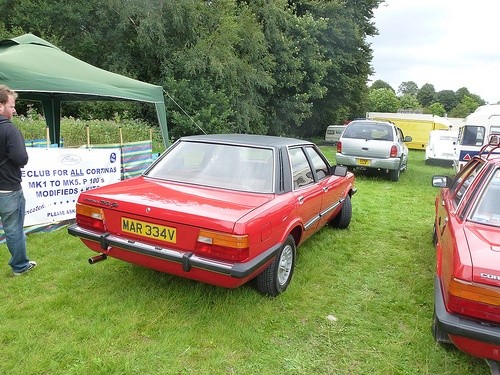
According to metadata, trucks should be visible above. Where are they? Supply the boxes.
[365,111,454,151]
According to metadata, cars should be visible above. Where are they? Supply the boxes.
[431,134,500,364]
[66,134,358,297]
[425,129,457,167]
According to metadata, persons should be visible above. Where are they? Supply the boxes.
[0,85,38,276]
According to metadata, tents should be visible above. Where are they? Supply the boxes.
[0,33,169,151]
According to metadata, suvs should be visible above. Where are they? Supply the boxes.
[336,118,412,182]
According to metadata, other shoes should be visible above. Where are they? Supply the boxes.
[14,262,37,275]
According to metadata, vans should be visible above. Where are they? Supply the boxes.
[325,124,347,142]
[452,99,500,190]
